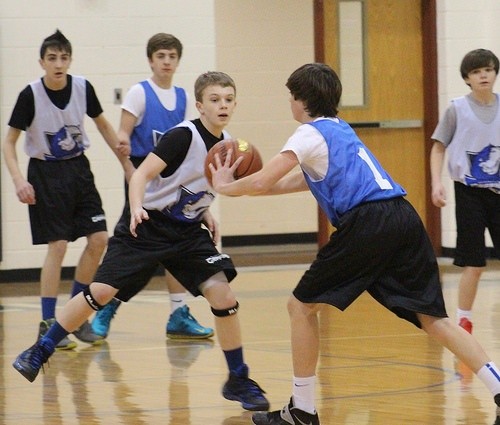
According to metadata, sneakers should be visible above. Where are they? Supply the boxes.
[12,337,56,384]
[72,319,104,345]
[223,364,270,411]
[251,395,320,425]
[37,319,78,349]
[167,305,214,338]
[492,393,500,425]
[91,297,121,337]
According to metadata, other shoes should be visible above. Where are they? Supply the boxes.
[459,317,472,334]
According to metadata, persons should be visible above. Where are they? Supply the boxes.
[90,33,215,339]
[4,30,137,353]
[208,61,500,425]
[12,72,269,411]
[430,49,500,334]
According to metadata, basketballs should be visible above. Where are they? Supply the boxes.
[205,137,264,193]
